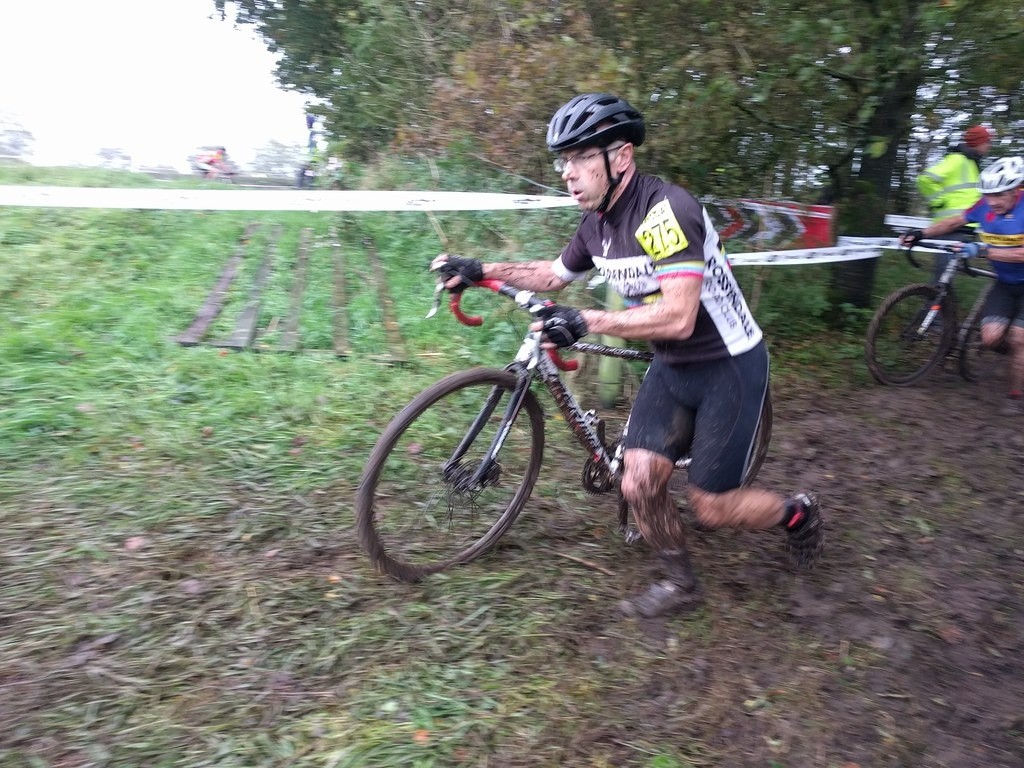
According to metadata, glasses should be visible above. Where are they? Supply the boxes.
[553,145,622,172]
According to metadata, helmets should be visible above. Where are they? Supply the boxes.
[546,90,645,151]
[976,156,1023,194]
[967,125,993,146]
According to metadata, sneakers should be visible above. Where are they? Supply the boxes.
[788,488,825,574]
[618,574,706,618]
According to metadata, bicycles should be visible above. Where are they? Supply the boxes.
[353,249,774,590]
[853,225,1011,391]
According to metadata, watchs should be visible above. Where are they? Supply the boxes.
[576,315,589,338]
[978,246,987,260]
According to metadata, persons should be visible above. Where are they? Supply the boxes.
[899,154,1024,396]
[430,93,826,622]
[213,146,233,175]
[914,127,995,328]
